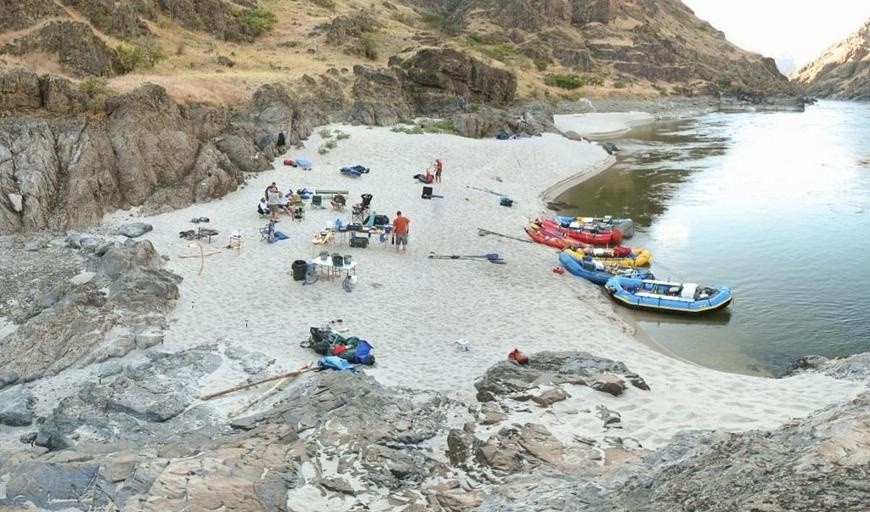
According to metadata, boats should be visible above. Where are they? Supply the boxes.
[523,213,733,315]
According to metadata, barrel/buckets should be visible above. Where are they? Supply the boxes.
[333,256,343,267]
[344,255,352,264]
[321,252,327,260]
[331,253,339,261]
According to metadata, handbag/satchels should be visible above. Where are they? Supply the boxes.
[392,235,394,245]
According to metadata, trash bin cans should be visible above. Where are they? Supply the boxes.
[421,187,432,199]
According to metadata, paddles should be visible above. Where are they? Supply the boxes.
[429,251,507,264]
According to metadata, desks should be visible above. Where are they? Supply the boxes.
[311,255,357,282]
[312,225,396,253]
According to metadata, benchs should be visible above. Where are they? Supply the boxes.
[315,189,349,199]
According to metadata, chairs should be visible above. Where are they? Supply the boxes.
[311,195,322,208]
[333,195,344,210]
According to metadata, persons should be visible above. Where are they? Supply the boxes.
[331,191,345,210]
[434,158,443,182]
[390,211,410,252]
[259,181,296,219]
